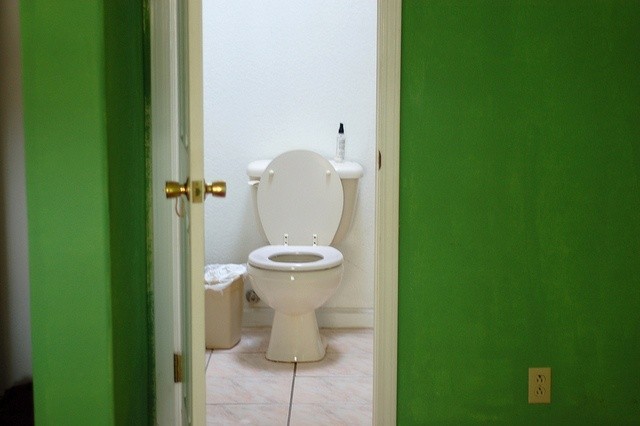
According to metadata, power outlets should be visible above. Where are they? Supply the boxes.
[528,367,551,404]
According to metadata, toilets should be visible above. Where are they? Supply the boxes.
[245,148,365,364]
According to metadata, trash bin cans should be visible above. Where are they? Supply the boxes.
[205,264,248,349]
